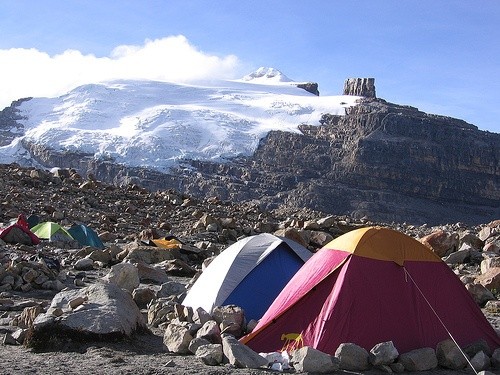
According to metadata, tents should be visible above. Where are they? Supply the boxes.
[237,225,500,360]
[0,215,107,252]
[180,231,316,330]
